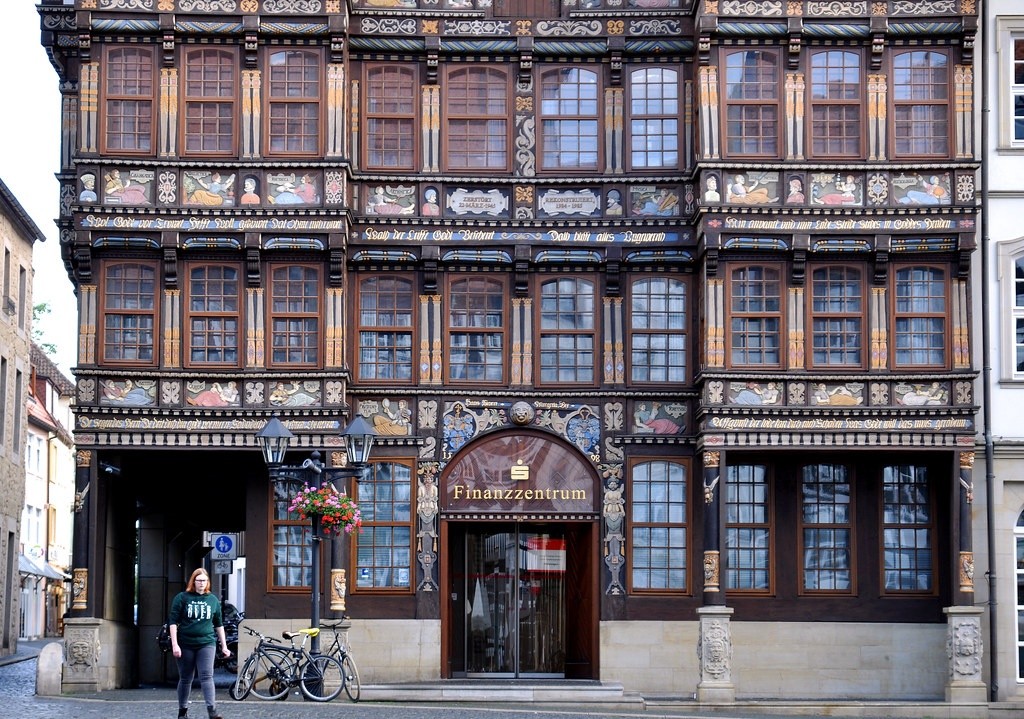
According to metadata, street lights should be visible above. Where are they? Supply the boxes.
[255,414,378,701]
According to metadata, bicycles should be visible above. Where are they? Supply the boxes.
[214,615,361,703]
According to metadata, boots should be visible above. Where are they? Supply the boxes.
[178,707,191,719]
[207,706,224,719]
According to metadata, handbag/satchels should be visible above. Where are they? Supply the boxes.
[158,623,175,653]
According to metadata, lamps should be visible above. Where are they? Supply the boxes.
[255,413,294,485]
[340,414,378,482]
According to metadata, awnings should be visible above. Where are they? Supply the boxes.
[18,555,72,580]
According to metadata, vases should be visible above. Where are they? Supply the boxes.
[317,516,333,539]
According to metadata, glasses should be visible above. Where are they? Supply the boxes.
[196,579,208,582]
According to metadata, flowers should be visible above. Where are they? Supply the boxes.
[287,481,364,536]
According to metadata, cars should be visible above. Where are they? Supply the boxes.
[219,599,245,654]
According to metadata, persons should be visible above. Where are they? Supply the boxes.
[169,568,231,719]
